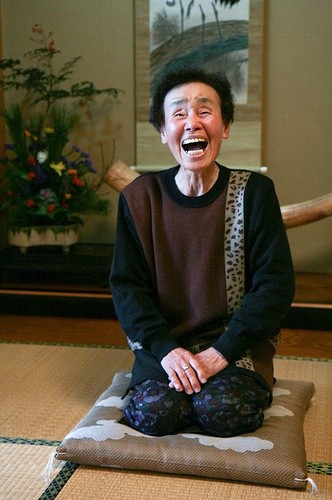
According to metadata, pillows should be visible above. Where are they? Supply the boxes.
[52,370,318,490]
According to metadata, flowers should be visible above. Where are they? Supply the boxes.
[0,21,127,223]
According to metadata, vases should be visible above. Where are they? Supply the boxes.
[7,221,82,254]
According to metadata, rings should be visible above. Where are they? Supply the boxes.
[183,366,193,372]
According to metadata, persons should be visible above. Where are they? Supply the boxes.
[109,68,297,439]
